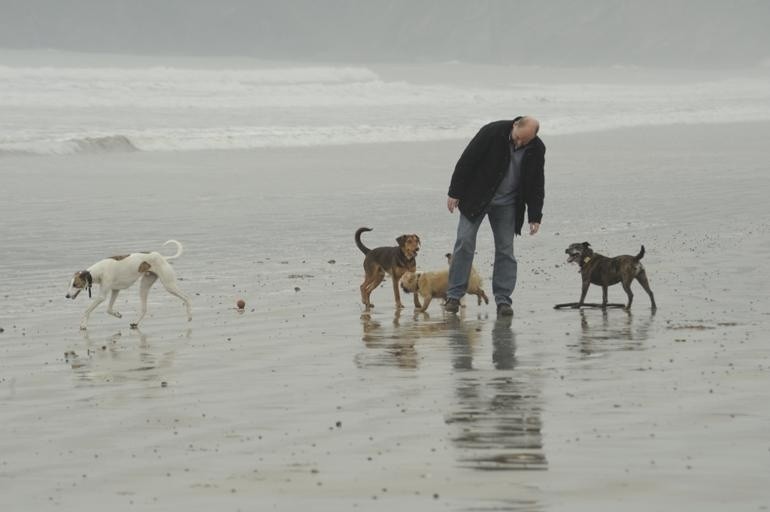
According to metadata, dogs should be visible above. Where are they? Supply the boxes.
[66,240,193,330]
[565,242,656,309]
[401,253,488,312]
[355,228,421,308]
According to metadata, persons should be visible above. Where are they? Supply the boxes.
[444,116,545,315]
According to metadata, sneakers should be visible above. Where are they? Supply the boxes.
[497,303,513,316]
[445,298,459,312]
